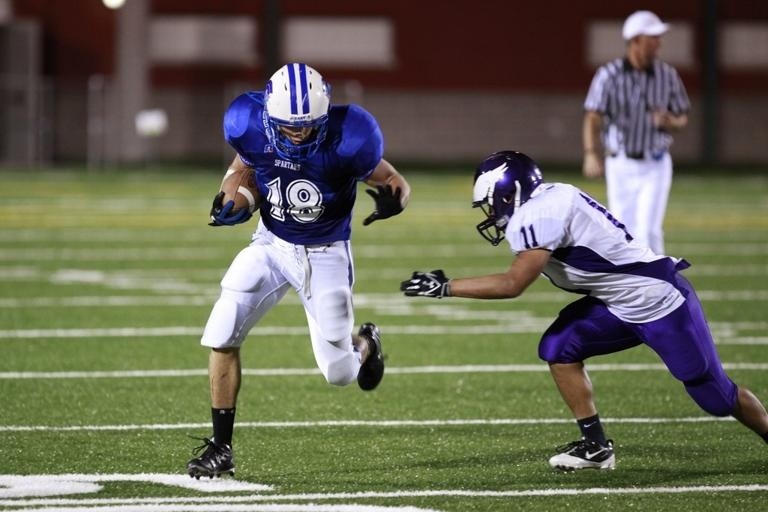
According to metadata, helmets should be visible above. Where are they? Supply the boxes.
[265,63,329,144]
[472,151,542,232]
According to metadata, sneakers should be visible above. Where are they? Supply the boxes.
[549,440,615,469]
[187,441,234,479]
[357,321,384,390]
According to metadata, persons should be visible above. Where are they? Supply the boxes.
[398,145,767,475]
[186,59,414,479]
[580,8,695,256]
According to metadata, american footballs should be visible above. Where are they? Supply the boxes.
[221,168,261,215]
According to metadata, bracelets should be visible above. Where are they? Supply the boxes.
[584,147,600,155]
[235,184,255,216]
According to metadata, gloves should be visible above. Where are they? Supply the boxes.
[400,270,450,299]
[210,191,252,224]
[363,183,403,226]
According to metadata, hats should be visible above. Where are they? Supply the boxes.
[622,11,669,40]
